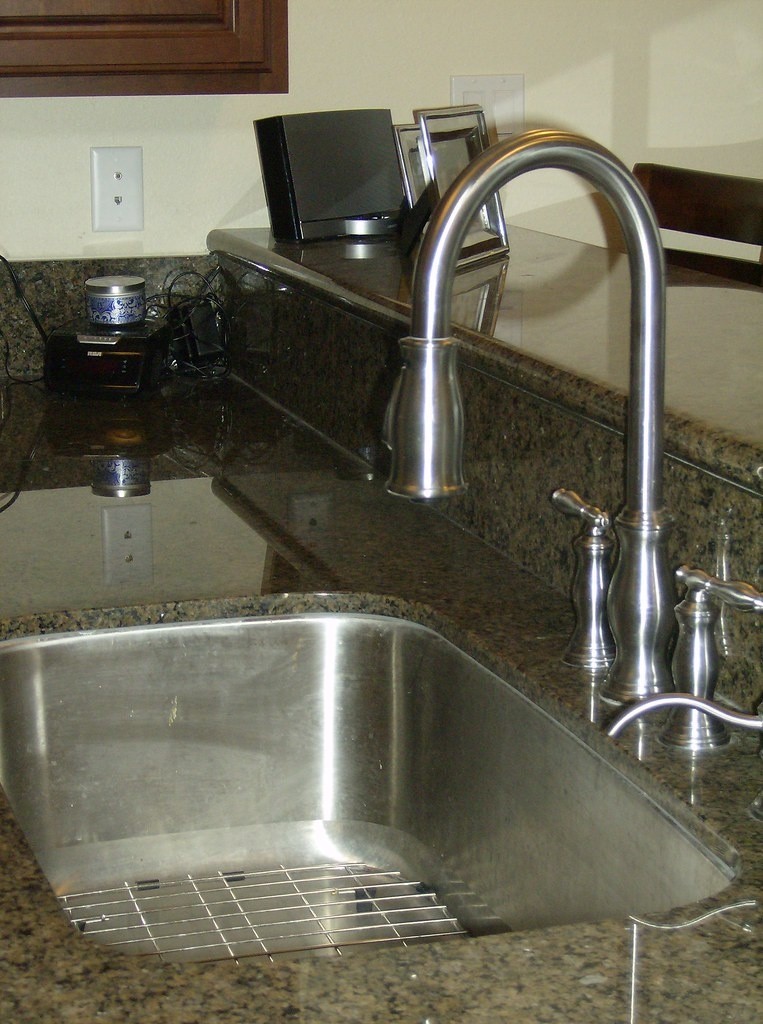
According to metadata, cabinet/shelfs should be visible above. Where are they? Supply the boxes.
[0,0,290,96]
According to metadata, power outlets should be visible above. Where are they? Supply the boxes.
[90,146,145,233]
[99,503,155,589]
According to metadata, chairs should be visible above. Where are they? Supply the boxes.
[631,161,763,287]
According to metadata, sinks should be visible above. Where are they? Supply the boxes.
[0,583,749,978]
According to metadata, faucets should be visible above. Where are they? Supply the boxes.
[380,122,680,707]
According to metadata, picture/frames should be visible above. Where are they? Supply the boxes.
[412,103,511,271]
[392,123,425,235]
[451,255,509,338]
